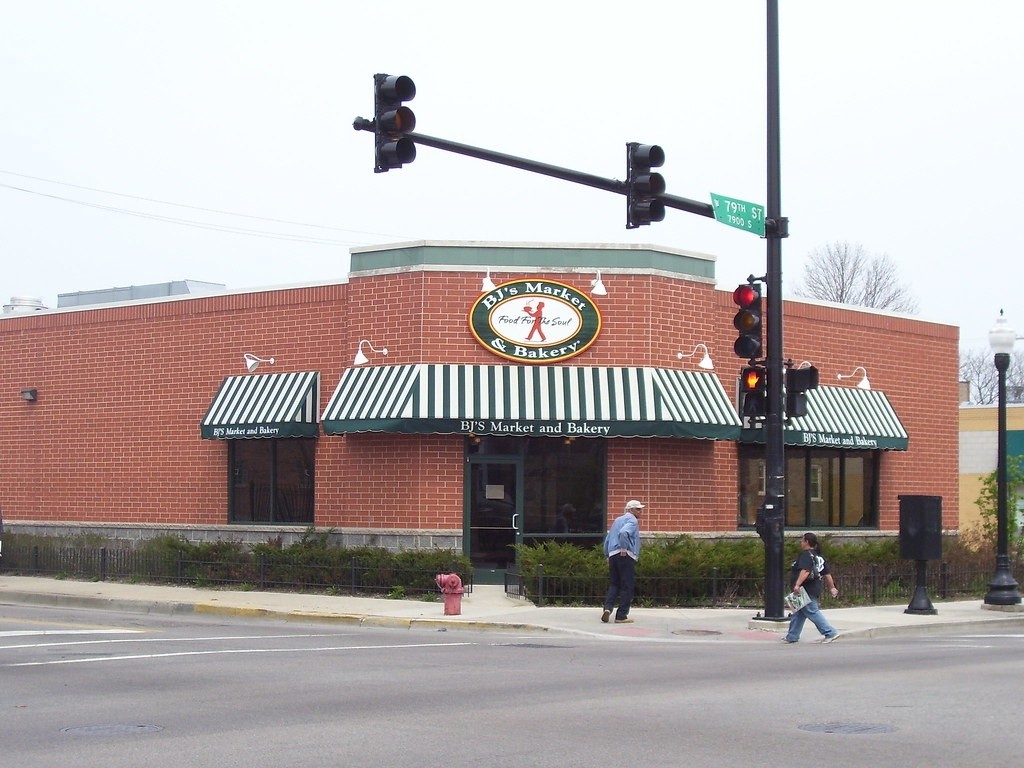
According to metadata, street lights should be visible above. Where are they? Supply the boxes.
[981,308,1024,610]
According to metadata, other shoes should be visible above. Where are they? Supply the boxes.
[780,638,793,643]
[822,634,839,643]
[615,618,634,623]
[602,610,611,622]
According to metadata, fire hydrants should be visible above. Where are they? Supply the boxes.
[436,573,465,616]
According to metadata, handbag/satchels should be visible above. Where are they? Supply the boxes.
[797,551,819,582]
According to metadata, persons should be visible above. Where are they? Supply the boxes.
[601,500,645,623]
[780,533,841,644]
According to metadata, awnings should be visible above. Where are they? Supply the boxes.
[200,372,321,440]
[737,376,909,451]
[320,365,745,437]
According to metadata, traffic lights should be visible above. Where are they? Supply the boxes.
[739,367,766,416]
[785,365,819,418]
[373,74,417,173]
[627,141,666,229]
[733,284,763,359]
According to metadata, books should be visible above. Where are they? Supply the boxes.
[784,586,812,614]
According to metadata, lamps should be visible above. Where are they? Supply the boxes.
[480,267,497,292]
[837,366,871,391]
[677,344,714,371]
[22,389,37,401]
[353,339,388,366]
[591,270,608,296]
[244,353,274,373]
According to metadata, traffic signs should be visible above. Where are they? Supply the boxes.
[710,192,765,235]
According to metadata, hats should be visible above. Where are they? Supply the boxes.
[626,500,645,509]
